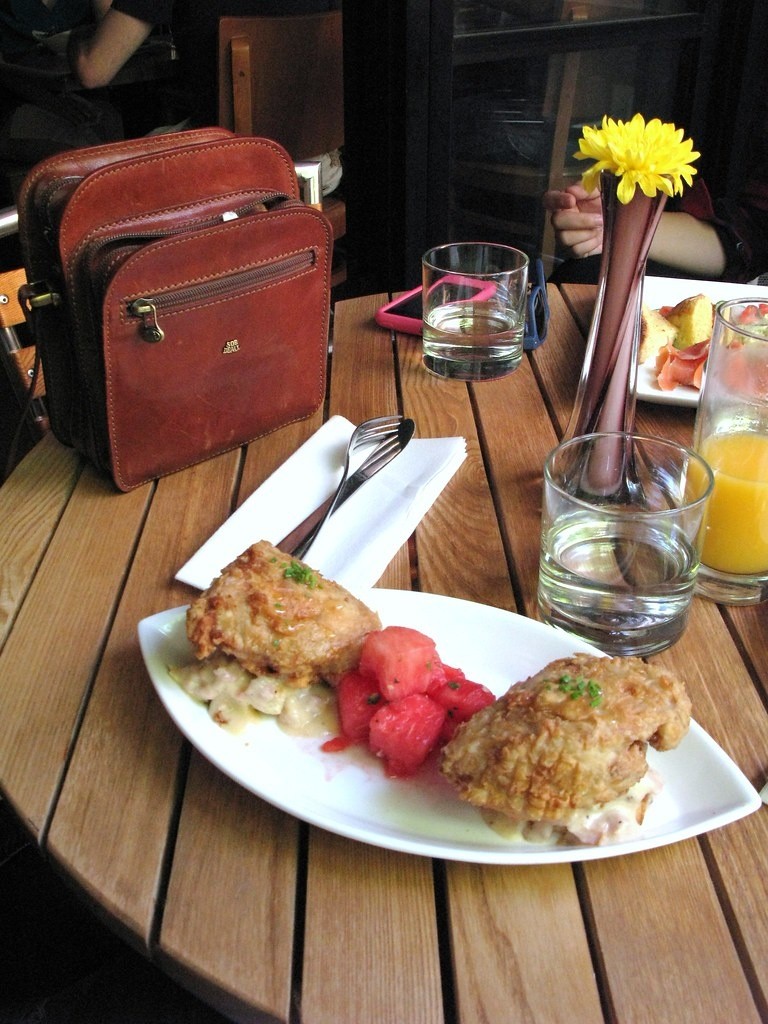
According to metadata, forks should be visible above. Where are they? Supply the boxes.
[291,413,402,561]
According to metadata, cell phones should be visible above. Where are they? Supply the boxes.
[376,273,496,339]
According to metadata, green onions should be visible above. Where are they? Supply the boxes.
[544,674,603,708]
[270,556,324,590]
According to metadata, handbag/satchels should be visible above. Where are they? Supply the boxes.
[19,128,330,492]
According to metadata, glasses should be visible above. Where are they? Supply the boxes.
[523,258,550,348]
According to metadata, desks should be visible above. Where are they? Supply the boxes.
[0,278,768,1024]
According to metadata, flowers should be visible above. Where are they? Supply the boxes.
[568,109,700,202]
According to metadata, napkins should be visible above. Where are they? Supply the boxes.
[175,414,469,596]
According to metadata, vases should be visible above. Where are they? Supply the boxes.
[554,170,671,495]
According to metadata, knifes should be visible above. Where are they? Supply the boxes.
[276,418,415,558]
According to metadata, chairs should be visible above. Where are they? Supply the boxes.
[0,161,322,443]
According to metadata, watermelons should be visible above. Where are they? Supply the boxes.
[335,625,500,780]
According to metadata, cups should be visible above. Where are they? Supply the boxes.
[662,295,768,606]
[532,431,715,658]
[420,241,530,382]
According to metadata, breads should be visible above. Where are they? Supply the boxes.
[635,294,736,365]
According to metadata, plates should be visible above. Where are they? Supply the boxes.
[136,588,763,865]
[631,275,768,407]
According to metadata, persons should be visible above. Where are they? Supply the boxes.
[543,176,768,285]
[69,0,201,142]
